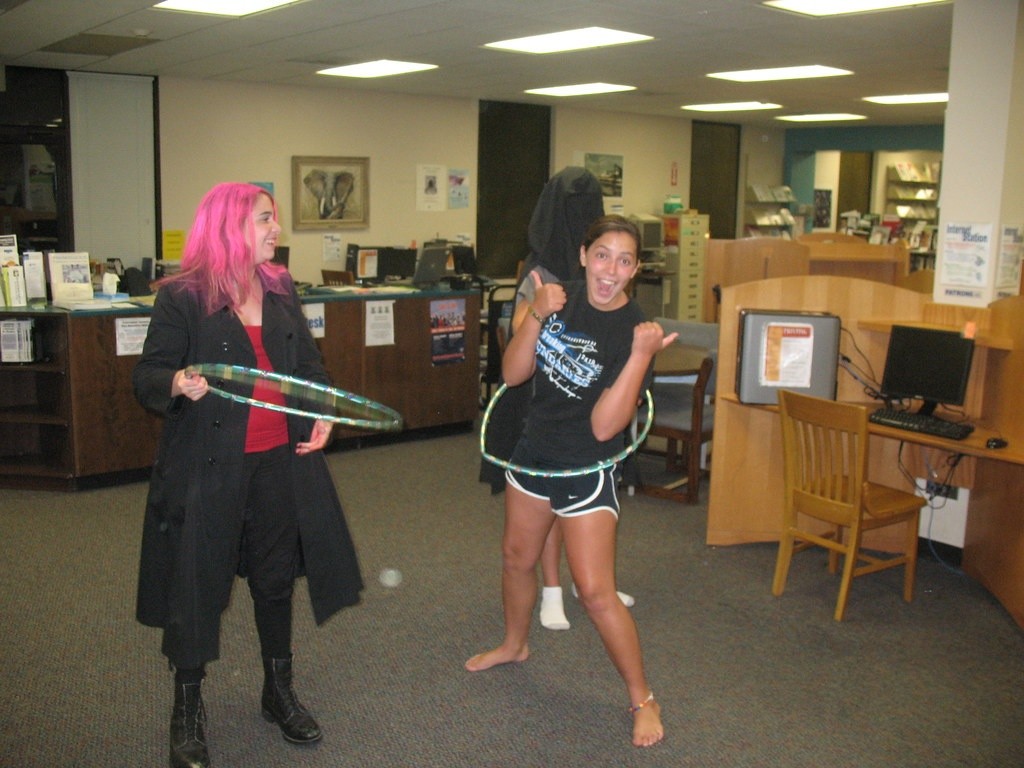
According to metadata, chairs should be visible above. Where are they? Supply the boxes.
[480,284,518,412]
[629,355,714,505]
[773,387,926,623]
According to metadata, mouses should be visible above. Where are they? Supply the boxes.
[986,437,1008,449]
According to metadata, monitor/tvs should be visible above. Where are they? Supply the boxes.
[344,239,477,286]
[880,324,974,418]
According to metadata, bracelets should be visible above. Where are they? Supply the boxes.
[528,306,545,323]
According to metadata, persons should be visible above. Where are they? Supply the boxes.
[465,215,678,746]
[136,183,365,768]
[517,166,635,631]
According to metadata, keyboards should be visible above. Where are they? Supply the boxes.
[870,408,975,440]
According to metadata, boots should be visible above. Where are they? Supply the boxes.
[262,654,323,744]
[168,672,210,768]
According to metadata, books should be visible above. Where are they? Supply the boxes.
[750,161,941,273]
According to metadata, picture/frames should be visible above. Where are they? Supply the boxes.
[290,155,368,231]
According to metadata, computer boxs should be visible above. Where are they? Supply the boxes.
[735,309,841,405]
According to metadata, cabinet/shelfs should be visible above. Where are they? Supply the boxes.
[886,165,940,219]
[707,274,1024,625]
[652,214,710,324]
[0,363,74,480]
[744,189,797,240]
